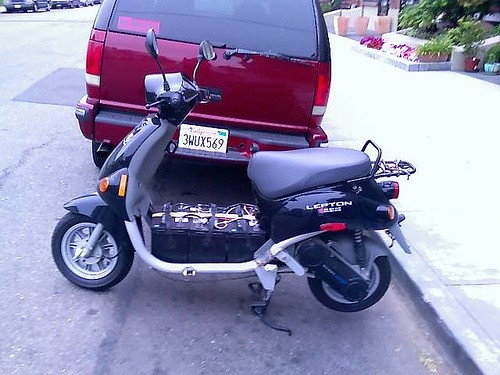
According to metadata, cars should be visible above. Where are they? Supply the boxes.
[3,0,100,14]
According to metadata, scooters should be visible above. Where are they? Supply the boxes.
[51,30,417,335]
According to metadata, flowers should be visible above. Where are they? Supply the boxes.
[360,36,416,60]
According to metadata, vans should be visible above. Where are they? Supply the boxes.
[77,1,333,170]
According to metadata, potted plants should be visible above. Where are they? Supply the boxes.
[447,15,485,72]
[484,42,500,76]
[414,36,456,62]
